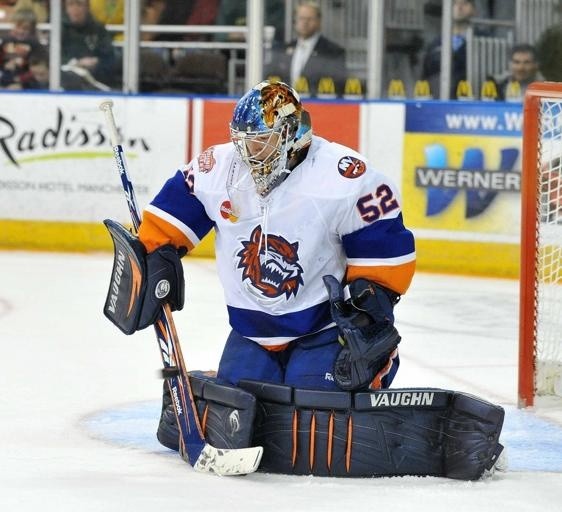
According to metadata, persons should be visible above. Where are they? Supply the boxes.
[274,1,346,95]
[137,80,416,392]
[422,0,488,99]
[500,44,547,101]
[0,0,228,95]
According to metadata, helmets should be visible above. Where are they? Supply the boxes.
[229,80,312,157]
